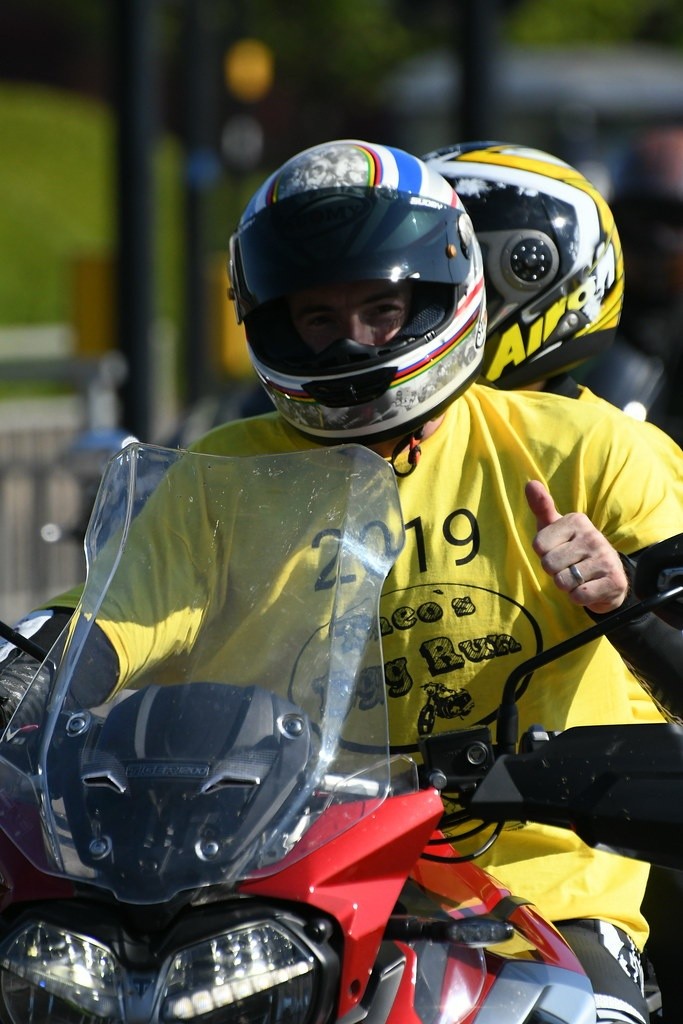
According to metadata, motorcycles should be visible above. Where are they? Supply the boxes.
[0,437,680,1024]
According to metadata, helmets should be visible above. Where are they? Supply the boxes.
[414,140,627,392]
[225,137,490,449]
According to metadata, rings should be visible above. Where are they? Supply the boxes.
[568,564,584,586]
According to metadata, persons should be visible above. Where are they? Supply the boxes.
[416,144,625,409]
[4,141,683,1023]
